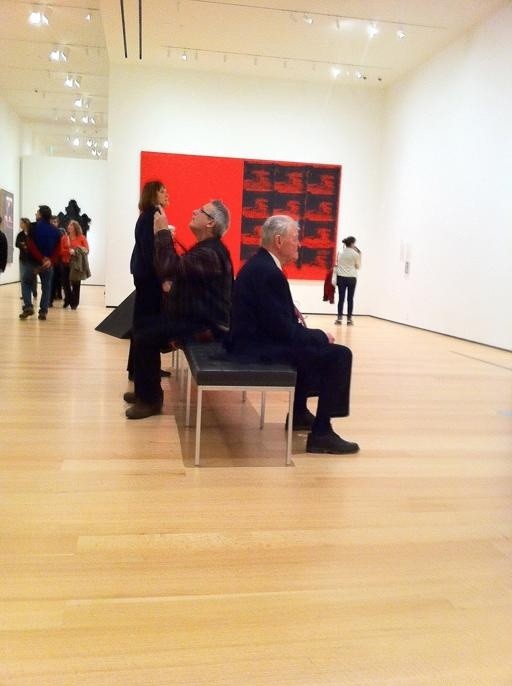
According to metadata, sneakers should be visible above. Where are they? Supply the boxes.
[19,310,34,318]
[347,320,353,325]
[335,319,342,324]
[38,313,47,320]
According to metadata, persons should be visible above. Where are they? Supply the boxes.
[224,214,359,455]
[20,205,63,321]
[61,220,89,310]
[335,236,361,325]
[123,200,234,419]
[15,218,38,299]
[63,199,88,238]
[126,181,172,381]
[0,216,8,275]
[49,215,68,300]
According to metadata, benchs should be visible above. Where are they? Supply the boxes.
[172,344,298,467]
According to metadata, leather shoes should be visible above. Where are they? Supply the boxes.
[126,392,163,419]
[123,392,138,402]
[160,370,171,377]
[128,372,135,380]
[307,427,358,455]
[285,410,316,430]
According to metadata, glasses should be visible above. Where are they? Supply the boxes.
[199,206,214,220]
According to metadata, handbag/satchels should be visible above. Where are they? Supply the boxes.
[332,251,339,288]
